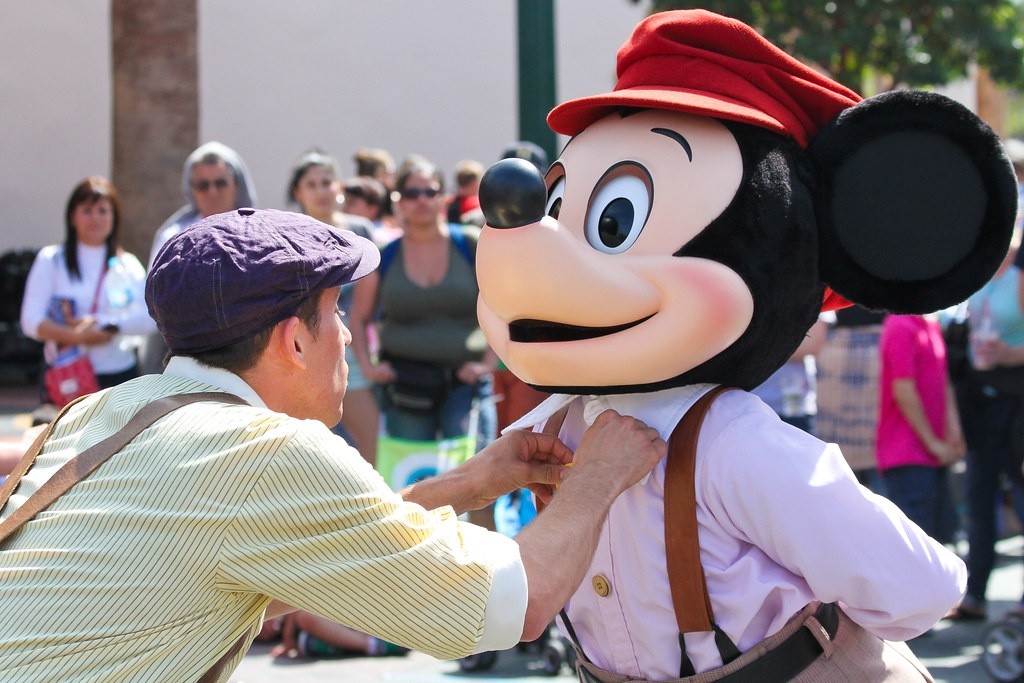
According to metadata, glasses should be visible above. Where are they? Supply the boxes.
[191,178,229,191]
[400,187,438,199]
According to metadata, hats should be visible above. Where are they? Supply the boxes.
[145,208,381,353]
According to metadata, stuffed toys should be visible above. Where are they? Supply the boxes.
[457,9,1018,683]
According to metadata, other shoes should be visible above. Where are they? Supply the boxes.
[940,604,986,621]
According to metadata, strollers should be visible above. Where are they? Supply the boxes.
[377,372,566,678]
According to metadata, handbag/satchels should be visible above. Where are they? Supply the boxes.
[46,355,99,409]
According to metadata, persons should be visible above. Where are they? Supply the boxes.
[0,139,1024,683]
